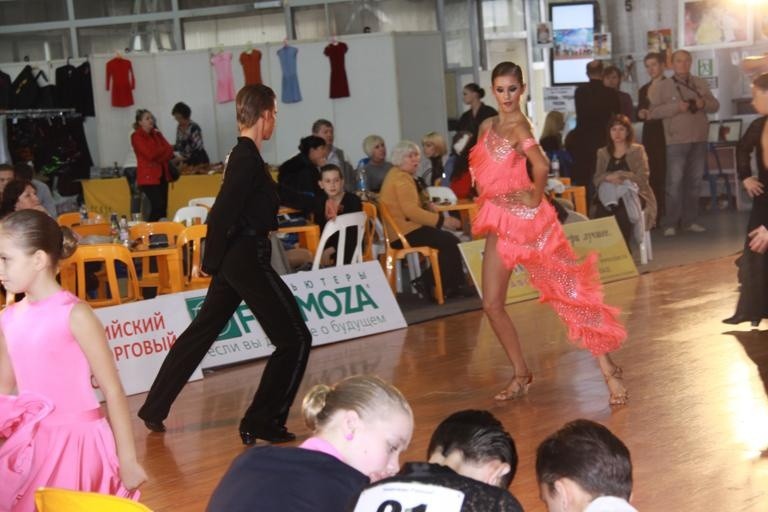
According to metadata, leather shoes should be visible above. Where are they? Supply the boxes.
[238,427,295,445]
[139,413,168,433]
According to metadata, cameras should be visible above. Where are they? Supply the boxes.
[684,98,698,115]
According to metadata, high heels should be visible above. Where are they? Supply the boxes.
[603,368,631,406]
[723,312,762,327]
[494,373,534,401]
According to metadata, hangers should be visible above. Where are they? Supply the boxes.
[82,53,90,71]
[64,55,74,72]
[219,43,225,56]
[244,40,253,55]
[331,33,337,46]
[113,48,121,60]
[283,37,288,48]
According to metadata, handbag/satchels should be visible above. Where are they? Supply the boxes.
[161,161,180,184]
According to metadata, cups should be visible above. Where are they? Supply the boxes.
[133,212,142,224]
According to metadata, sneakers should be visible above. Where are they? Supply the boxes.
[449,288,476,300]
[688,223,707,233]
[410,277,436,301]
[665,227,678,237]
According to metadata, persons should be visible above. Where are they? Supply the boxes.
[137,83,339,447]
[275,83,498,303]
[0,161,57,307]
[0,208,147,512]
[723,74,767,332]
[534,419,640,512]
[170,99,209,175]
[468,59,630,408]
[351,408,526,512]
[129,107,175,222]
[539,49,721,254]
[205,372,415,510]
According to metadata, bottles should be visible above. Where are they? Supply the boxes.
[356,159,368,193]
[110,213,128,247]
[79,203,88,226]
[551,153,560,177]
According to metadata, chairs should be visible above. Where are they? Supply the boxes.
[549,176,571,195]
[10,212,216,307]
[311,211,367,270]
[276,205,308,226]
[377,198,445,305]
[361,201,377,261]
[638,208,653,264]
[704,143,734,214]
